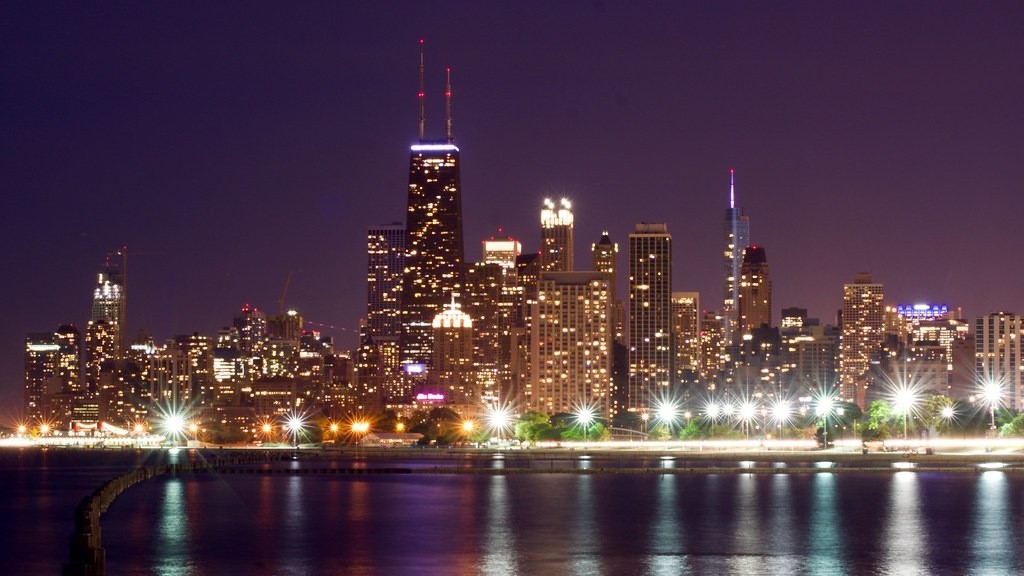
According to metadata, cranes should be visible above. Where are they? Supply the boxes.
[278,270,293,314]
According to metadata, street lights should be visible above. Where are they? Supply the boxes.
[582,412,586,445]
[987,383,996,445]
[20,414,367,451]
[496,416,501,447]
[775,405,783,442]
[655,398,761,445]
[901,392,909,447]
[820,399,828,450]
